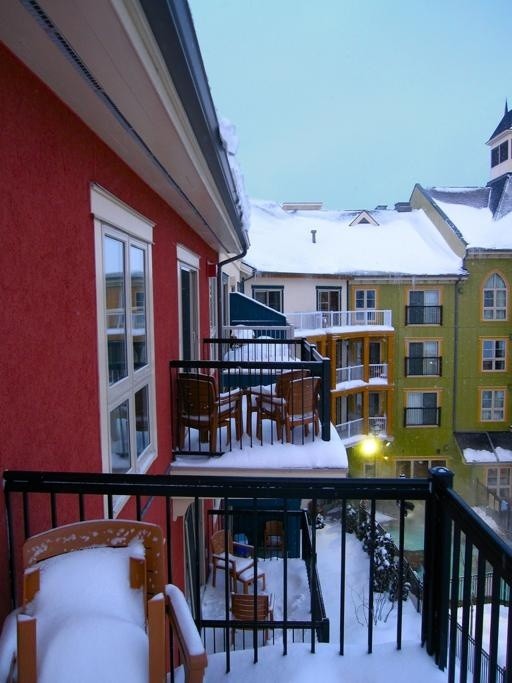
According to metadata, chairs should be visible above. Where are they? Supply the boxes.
[174,368,324,459]
[208,520,288,645]
[10,518,209,683]
